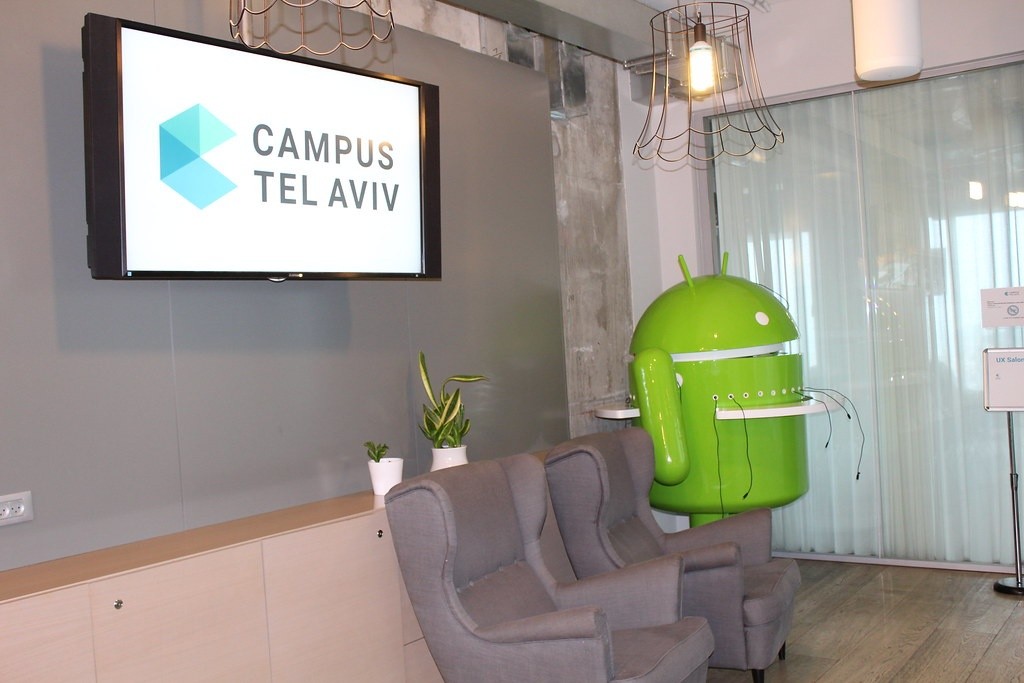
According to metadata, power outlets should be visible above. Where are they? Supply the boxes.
[0,491,34,528]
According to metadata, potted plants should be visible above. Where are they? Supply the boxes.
[417,349,490,473]
[363,441,404,496]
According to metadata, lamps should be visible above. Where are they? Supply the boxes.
[632,0,785,171]
[853,0,923,82]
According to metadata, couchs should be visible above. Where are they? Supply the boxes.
[383,452,716,683]
[545,427,802,683]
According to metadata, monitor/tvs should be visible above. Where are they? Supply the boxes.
[80,13,441,282]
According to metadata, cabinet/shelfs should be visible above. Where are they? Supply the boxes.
[0,491,443,683]
